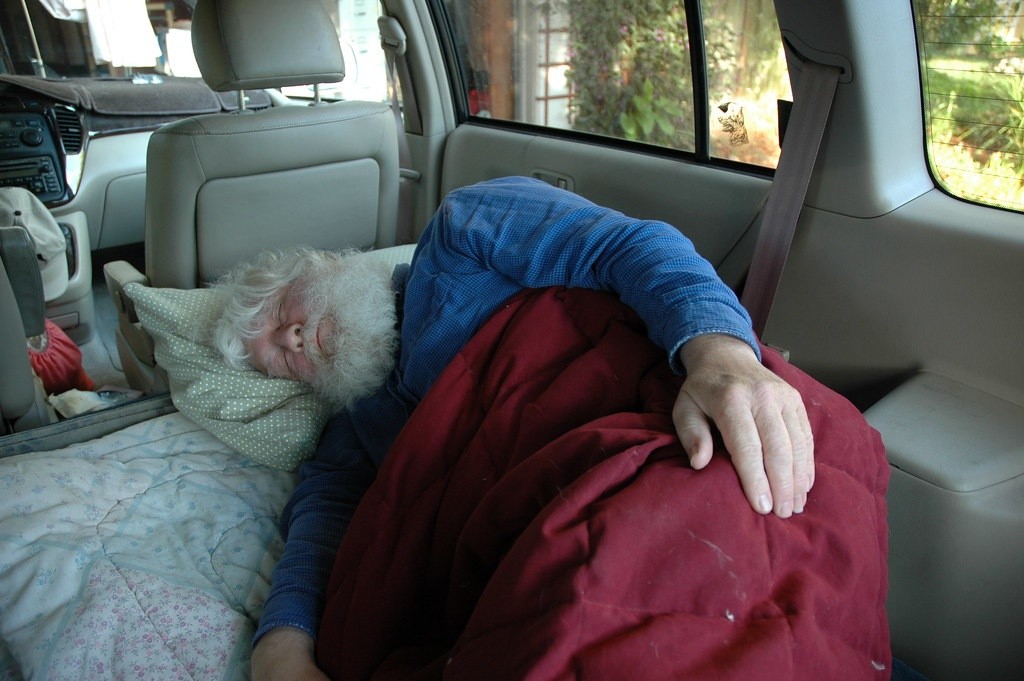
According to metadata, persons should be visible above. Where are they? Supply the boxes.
[213,175,890,681]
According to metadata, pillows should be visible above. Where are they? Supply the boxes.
[122,243,418,472]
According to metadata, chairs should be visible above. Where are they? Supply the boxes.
[103,0,400,399]
[0,226,59,439]
